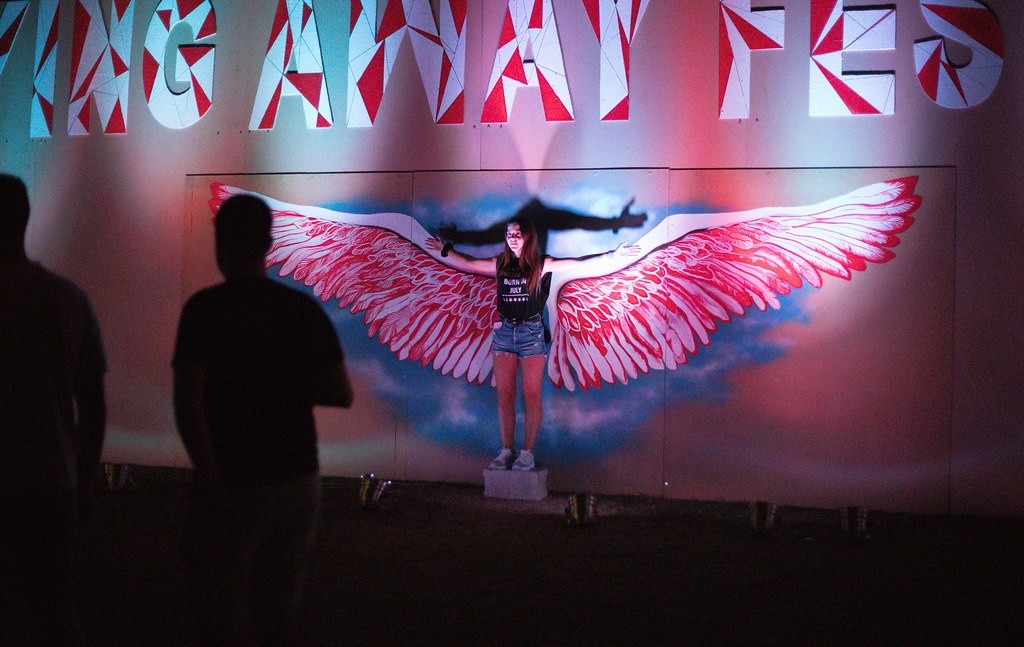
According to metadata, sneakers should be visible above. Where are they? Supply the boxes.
[488,446,515,470]
[512,449,535,470]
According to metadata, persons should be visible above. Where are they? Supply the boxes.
[0,172,108,647]
[170,195,354,647]
[424,216,641,471]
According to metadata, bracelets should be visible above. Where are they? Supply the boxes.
[441,243,454,257]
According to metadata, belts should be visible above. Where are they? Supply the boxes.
[498,314,539,325]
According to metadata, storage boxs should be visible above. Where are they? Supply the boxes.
[483,467,549,503]
